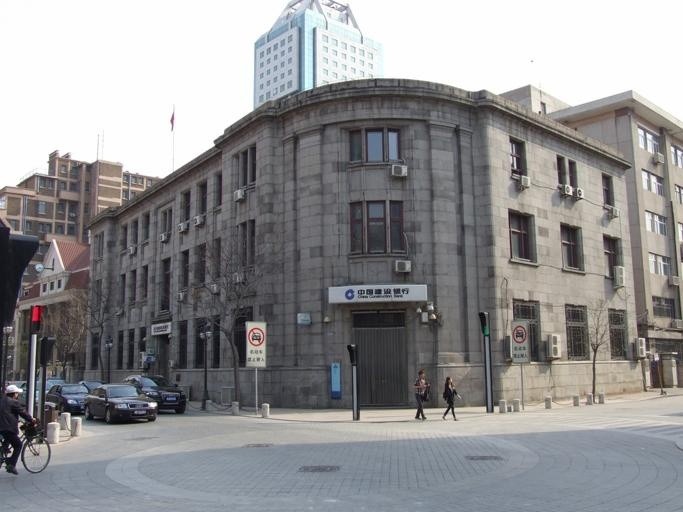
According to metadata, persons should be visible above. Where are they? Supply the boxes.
[411,370,431,420]
[0,384,36,474]
[440,374,462,421]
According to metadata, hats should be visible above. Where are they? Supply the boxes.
[3,371,187,425]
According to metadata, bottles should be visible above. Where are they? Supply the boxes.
[5,385,23,393]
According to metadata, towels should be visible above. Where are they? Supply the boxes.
[177,385,190,401]
[220,387,236,405]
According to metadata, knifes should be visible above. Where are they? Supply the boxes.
[560,185,584,202]
[667,275,680,286]
[137,351,148,369]
[391,164,407,178]
[608,207,619,219]
[158,215,205,243]
[209,271,245,295]
[167,359,176,368]
[233,189,244,202]
[671,319,682,329]
[612,265,625,291]
[653,152,664,165]
[395,260,411,273]
[518,175,530,191]
[175,291,185,302]
[546,334,561,362]
[633,337,646,360]
[126,246,134,255]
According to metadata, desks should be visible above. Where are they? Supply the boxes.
[198,320,213,413]
[103,337,113,384]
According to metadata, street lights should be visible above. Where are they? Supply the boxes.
[423,393,432,401]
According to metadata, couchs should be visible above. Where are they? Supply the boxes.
[346,344,357,365]
[478,311,490,337]
[39,336,56,366]
[29,303,42,335]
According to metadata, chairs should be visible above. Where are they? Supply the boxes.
[7,465,18,474]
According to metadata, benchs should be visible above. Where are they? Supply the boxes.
[169,112,175,131]
